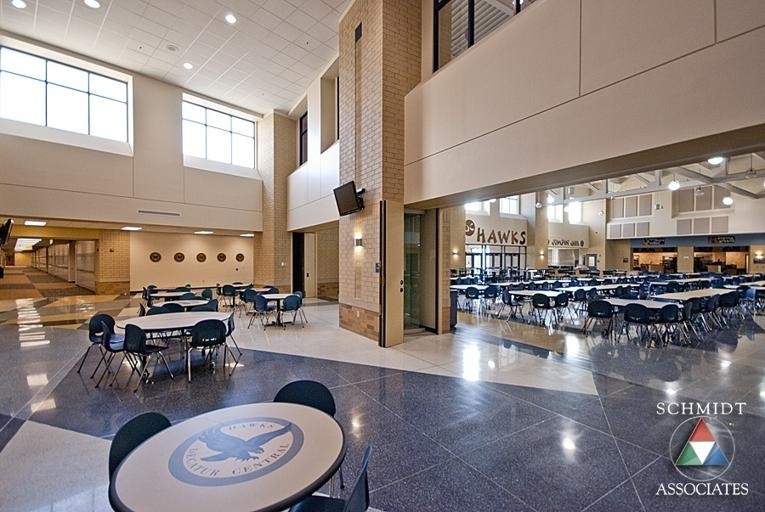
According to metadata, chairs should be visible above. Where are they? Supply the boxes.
[76,279,309,395]
[285,443,375,512]
[272,380,346,491]
[108,411,172,484]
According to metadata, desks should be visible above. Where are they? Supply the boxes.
[110,402,349,512]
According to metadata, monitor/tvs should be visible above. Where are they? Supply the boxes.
[333,181,364,217]
[0,217,14,245]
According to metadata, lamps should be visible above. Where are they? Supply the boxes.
[535,191,542,209]
[694,168,703,198]
[744,151,759,181]
[667,168,679,190]
